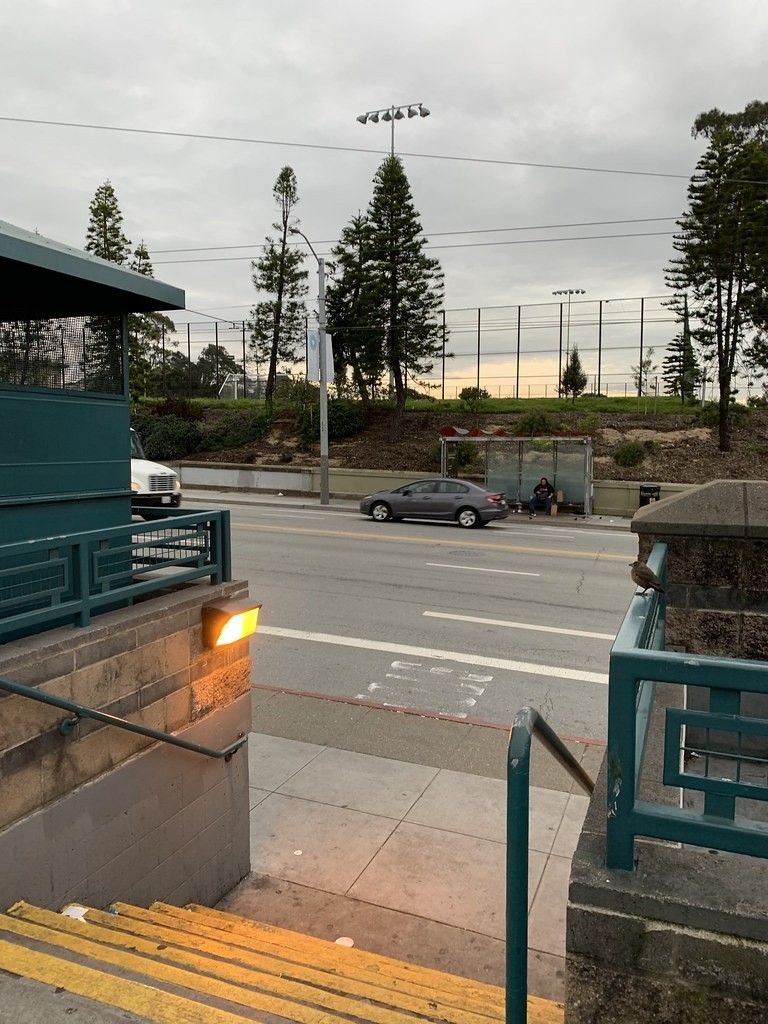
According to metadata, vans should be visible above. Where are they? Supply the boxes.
[129,426,182,521]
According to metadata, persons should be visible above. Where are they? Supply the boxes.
[529,478,555,517]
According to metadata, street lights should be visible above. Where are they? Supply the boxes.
[288,227,330,505]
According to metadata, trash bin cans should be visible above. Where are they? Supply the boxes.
[639,483,661,508]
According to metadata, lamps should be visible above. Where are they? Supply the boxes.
[202,597,263,652]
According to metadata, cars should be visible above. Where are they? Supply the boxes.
[359,478,511,528]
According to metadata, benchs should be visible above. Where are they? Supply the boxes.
[508,499,584,517]
